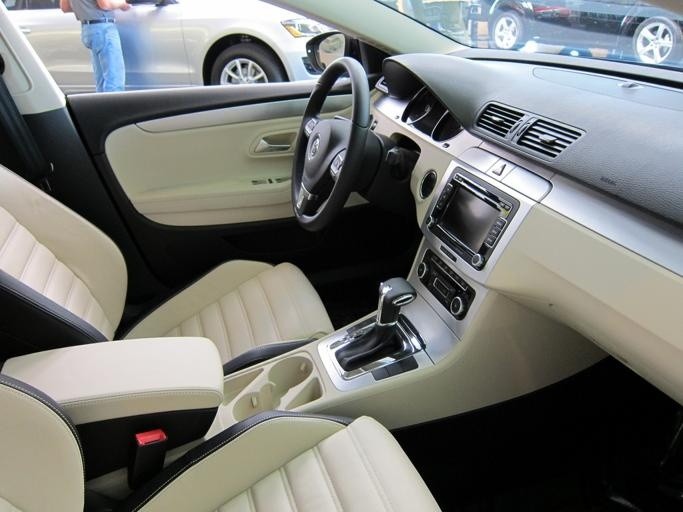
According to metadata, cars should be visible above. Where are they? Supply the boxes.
[468,0,681,67]
[2,1,343,86]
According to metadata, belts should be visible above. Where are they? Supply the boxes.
[83,19,114,24]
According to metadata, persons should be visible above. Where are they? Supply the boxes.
[58,1,132,93]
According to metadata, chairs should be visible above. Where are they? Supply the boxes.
[0,164,338,378]
[0,368,441,510]
